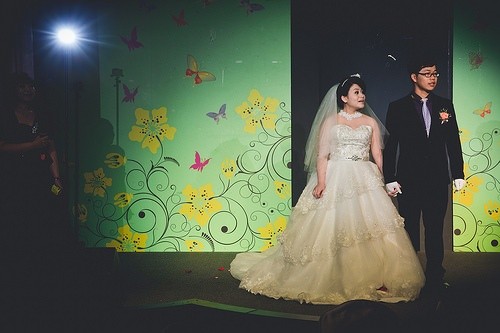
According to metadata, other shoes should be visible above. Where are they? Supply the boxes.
[429,277,450,287]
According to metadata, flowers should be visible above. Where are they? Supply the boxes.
[438,112,452,125]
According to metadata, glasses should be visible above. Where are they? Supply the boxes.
[418,73,439,78]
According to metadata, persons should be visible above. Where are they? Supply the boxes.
[384,57,466,289]
[232,73,426,306]
[0,71,64,196]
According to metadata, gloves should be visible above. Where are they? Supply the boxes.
[454,179,467,191]
[384,182,402,196]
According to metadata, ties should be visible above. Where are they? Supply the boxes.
[420,98,431,138]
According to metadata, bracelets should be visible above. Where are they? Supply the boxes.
[52,175,60,182]
